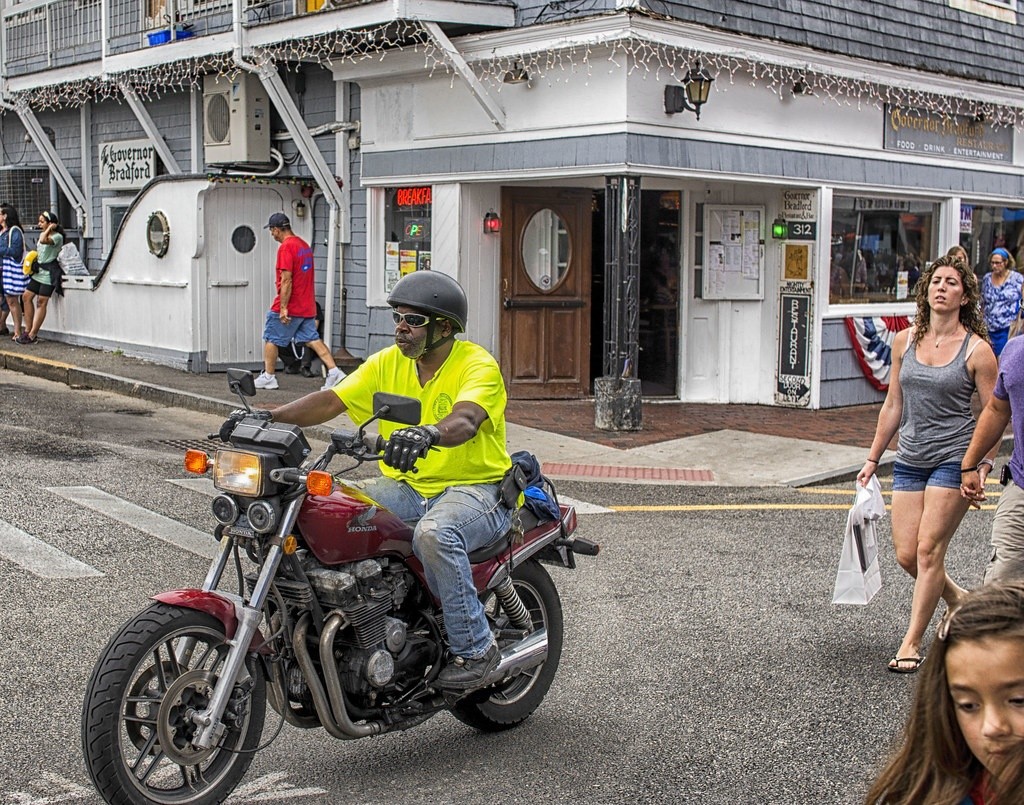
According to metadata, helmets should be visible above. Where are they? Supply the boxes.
[386,270,467,333]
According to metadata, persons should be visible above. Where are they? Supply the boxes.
[866,585,1024,805]
[857,256,1003,673]
[278,300,324,377]
[961,278,1024,586]
[221,270,526,690]
[830,247,925,294]
[947,246,978,288]
[15,211,63,346]
[253,213,347,391]
[0,203,23,341]
[980,247,1024,372]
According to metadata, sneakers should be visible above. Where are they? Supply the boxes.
[320,367,347,390]
[15,331,38,345]
[254,370,279,390]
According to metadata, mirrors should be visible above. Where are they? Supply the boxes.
[518,205,574,295]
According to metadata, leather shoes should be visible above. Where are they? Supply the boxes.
[437,644,502,689]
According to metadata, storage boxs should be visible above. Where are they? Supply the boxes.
[147,28,194,45]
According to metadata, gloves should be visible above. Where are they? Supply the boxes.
[383,424,440,473]
[220,408,274,444]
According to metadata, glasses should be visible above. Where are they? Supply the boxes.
[990,261,1004,267]
[391,310,446,328]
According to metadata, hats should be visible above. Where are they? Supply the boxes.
[263,213,290,229]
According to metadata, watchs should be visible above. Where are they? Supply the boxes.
[977,457,995,473]
[424,423,440,446]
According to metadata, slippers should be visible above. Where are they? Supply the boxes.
[887,656,926,673]
[936,610,948,629]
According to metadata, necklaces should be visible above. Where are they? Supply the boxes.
[931,335,945,349]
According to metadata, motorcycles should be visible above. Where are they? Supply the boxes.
[80,364,601,805]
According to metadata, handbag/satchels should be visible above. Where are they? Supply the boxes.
[831,473,887,605]
[56,242,90,276]
[2,226,31,296]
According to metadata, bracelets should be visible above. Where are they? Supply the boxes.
[961,466,977,472]
[867,458,879,464]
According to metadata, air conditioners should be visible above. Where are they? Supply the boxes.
[202,70,271,166]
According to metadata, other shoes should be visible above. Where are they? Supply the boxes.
[12,335,20,340]
[286,360,301,373]
[0,328,9,336]
[302,367,314,378]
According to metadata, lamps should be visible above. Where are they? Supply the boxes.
[503,62,528,84]
[791,70,813,95]
[664,60,715,120]
[968,114,984,125]
